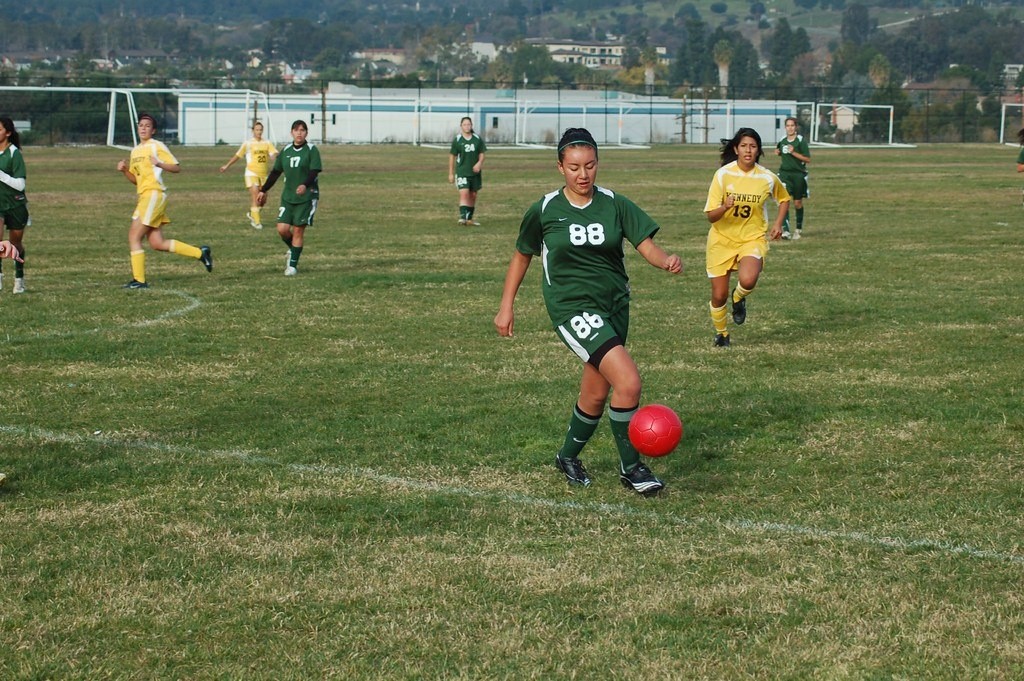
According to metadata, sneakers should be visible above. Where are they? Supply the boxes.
[732,288,746,325]
[458,219,466,225]
[793,229,802,239]
[620,460,664,494]
[199,246,211,272]
[13,283,25,294]
[285,258,297,276]
[782,231,791,239]
[287,248,291,253]
[0,275,3,290]
[466,220,480,226]
[124,279,147,288]
[714,333,730,347]
[247,212,263,229]
[554,448,592,489]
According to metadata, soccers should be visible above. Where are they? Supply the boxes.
[628,404,682,457]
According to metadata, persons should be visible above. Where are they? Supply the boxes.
[494,128,684,495]
[117,115,212,290]
[775,118,811,240]
[0,115,29,292]
[219,122,279,229]
[257,120,322,276]
[704,128,790,346]
[1017,128,1024,206]
[448,117,487,226]
